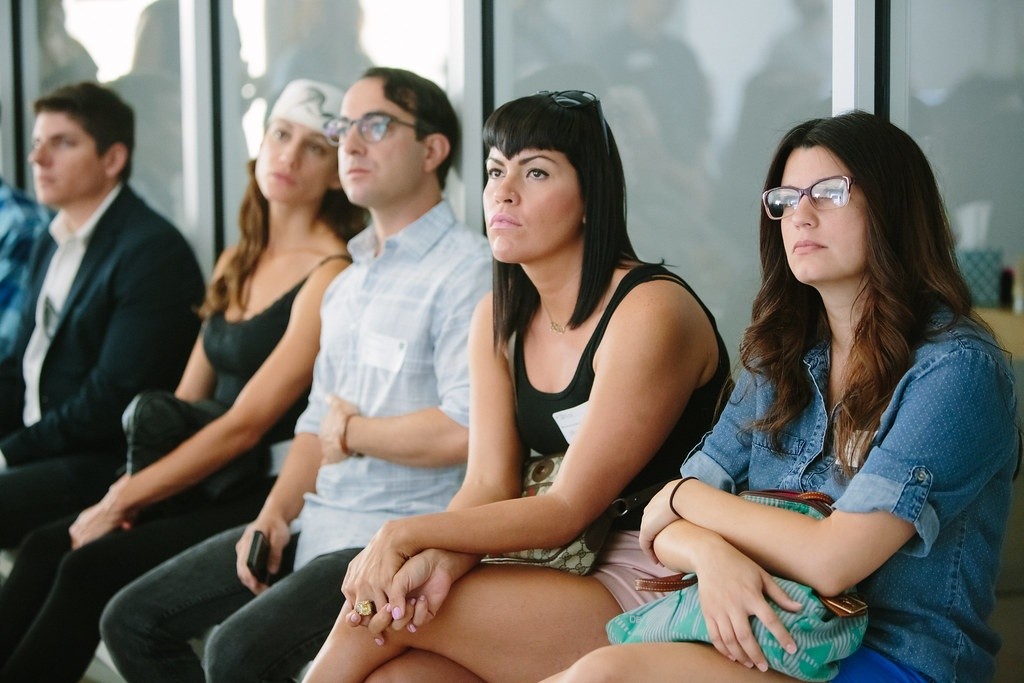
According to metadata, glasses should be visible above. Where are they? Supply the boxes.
[322,111,415,147]
[762,175,858,221]
[536,90,611,155]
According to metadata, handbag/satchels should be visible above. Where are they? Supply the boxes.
[477,452,630,576]
[121,389,268,520]
[605,488,872,683]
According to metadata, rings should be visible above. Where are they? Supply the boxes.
[356,600,375,616]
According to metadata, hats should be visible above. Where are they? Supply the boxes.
[267,77,345,140]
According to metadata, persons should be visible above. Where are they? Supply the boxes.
[538,109,1020,683]
[301,93,734,683]
[0,79,365,683]
[1,83,206,550]
[99,67,494,683]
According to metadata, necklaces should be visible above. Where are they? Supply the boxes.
[543,305,569,333]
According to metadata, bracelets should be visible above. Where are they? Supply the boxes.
[669,476,699,519]
[340,414,363,457]
